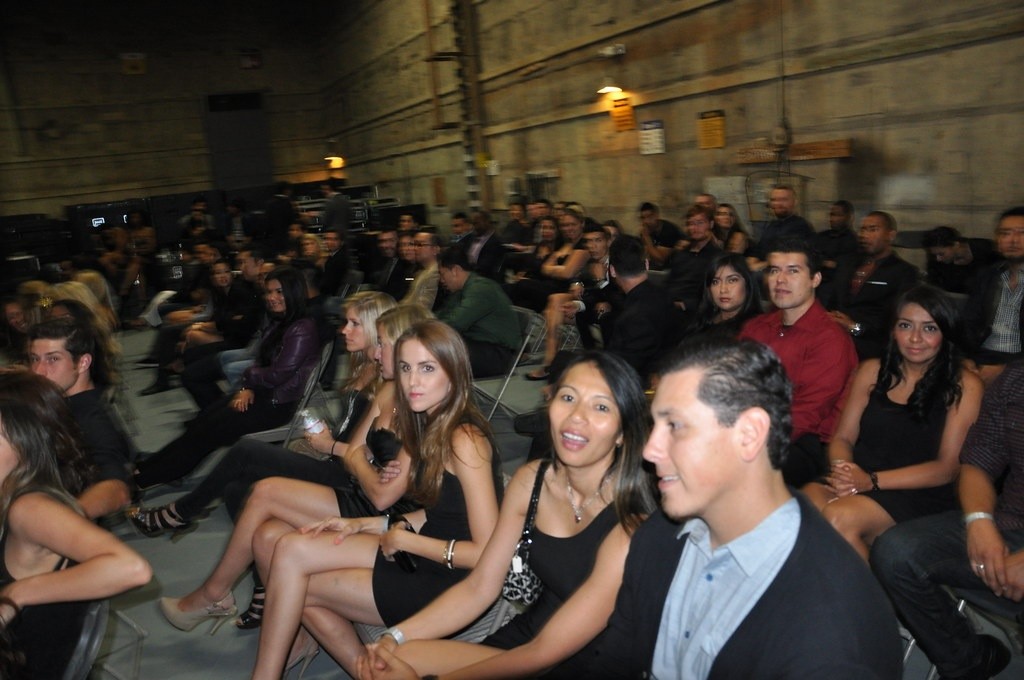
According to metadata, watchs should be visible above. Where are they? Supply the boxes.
[850,322,860,336]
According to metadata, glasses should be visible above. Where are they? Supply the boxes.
[413,240,435,248]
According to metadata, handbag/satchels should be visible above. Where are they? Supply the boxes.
[501,562,544,608]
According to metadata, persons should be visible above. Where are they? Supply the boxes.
[0,177,1024,679]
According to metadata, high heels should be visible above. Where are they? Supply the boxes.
[235,586,266,629]
[282,637,320,680]
[159,591,237,635]
[131,504,192,541]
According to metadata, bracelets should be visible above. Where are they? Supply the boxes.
[963,512,998,525]
[444,538,461,571]
[869,472,880,492]
[381,627,404,645]
[331,440,337,455]
[575,282,585,288]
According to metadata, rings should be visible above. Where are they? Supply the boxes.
[975,563,985,569]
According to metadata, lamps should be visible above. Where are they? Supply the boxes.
[323,150,341,160]
[597,76,624,95]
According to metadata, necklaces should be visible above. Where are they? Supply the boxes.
[565,462,618,523]
[393,400,399,414]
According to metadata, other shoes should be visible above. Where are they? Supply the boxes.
[133,466,167,492]
[939,633,1010,680]
[122,318,150,332]
[514,409,550,435]
[134,358,160,368]
[141,378,170,395]
[525,366,549,381]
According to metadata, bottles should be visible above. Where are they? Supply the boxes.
[302,410,324,434]
